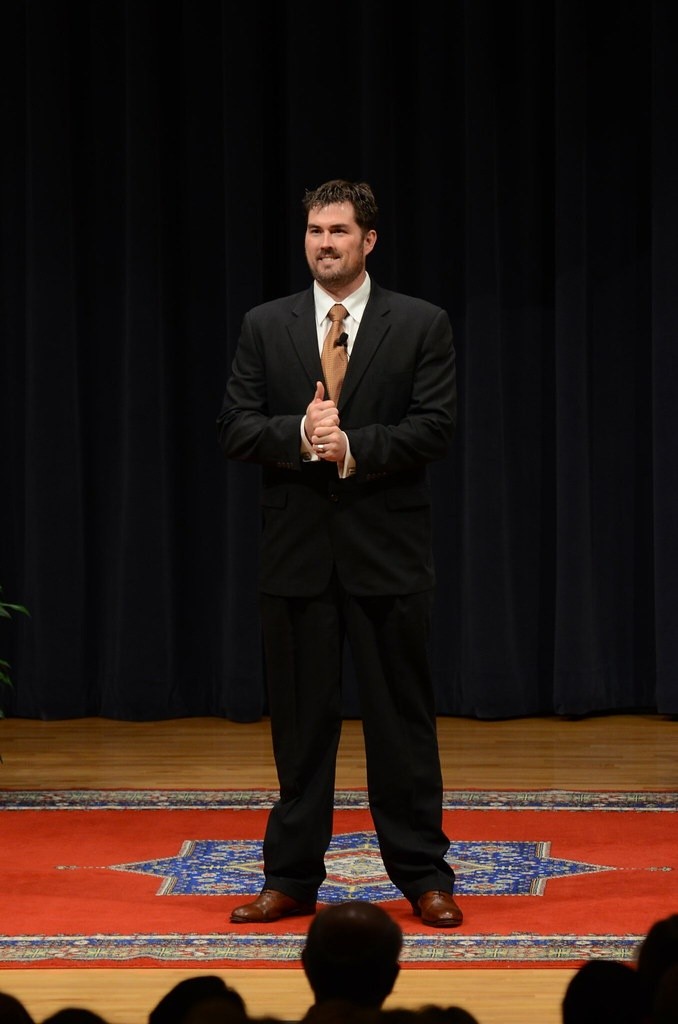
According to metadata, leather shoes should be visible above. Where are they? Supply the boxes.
[416,891,463,926]
[229,889,315,922]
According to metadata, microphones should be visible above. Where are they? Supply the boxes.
[333,332,348,348]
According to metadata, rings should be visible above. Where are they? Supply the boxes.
[318,444,324,452]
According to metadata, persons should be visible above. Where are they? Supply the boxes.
[217,181,464,925]
[561,914,678,1024]
[0,900,479,1024]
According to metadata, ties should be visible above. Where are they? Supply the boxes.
[320,304,349,408]
